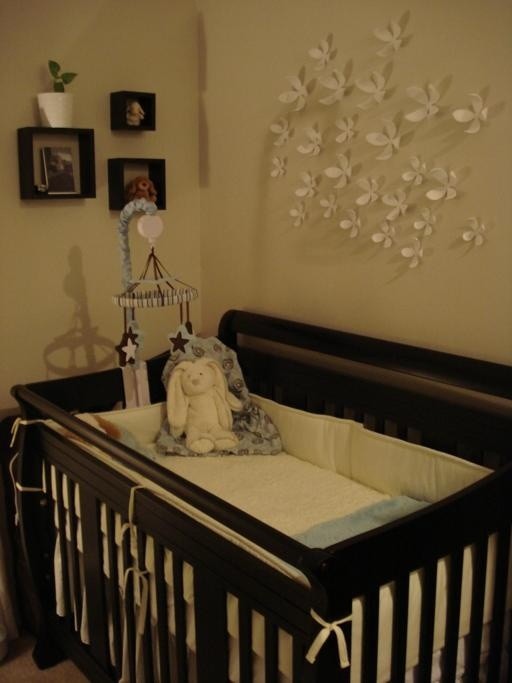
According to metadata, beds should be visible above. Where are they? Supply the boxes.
[10,307,512,682]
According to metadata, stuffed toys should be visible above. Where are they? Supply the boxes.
[126,100,146,127]
[130,176,158,202]
[112,244,199,368]
[166,357,243,454]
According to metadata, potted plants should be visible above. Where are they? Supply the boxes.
[38,58,78,126]
